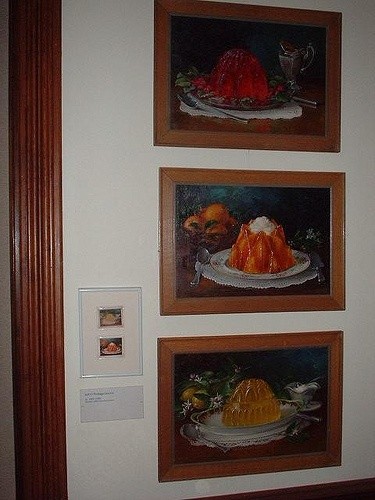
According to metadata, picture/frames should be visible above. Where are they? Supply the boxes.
[155,330,344,483]
[159,166,347,317]
[77,287,144,378]
[150,1,344,154]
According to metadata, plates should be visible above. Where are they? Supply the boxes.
[209,248,311,280]
[189,76,303,111]
[299,402,322,411]
[197,400,300,435]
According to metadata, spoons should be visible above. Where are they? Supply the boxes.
[191,248,210,285]
[180,425,231,453]
[177,92,250,123]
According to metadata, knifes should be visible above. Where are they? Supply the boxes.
[313,250,325,284]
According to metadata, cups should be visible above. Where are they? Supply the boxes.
[280,54,303,93]
[283,381,320,408]
